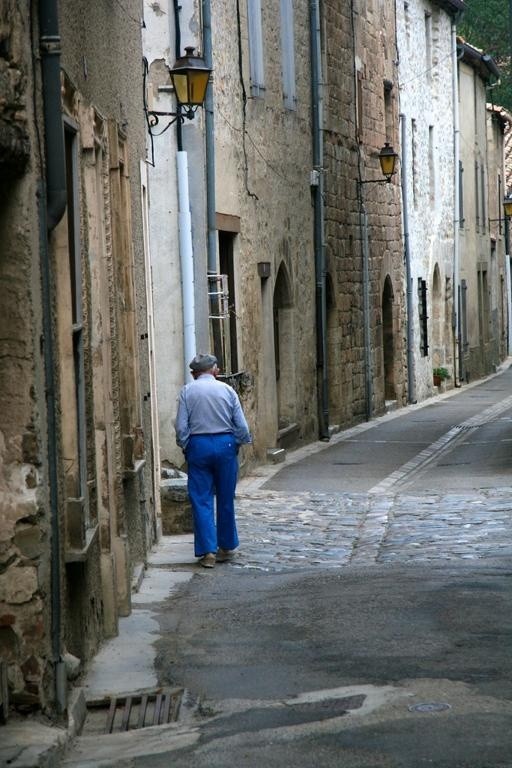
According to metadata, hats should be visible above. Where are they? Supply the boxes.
[188,352,218,371]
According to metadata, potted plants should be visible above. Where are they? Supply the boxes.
[433,367,450,385]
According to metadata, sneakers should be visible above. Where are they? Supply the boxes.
[215,549,238,562]
[199,557,218,569]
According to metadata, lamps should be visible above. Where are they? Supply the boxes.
[354,141,398,185]
[140,47,213,136]
[487,195,511,222]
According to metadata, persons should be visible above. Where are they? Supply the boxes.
[174,353,252,568]
